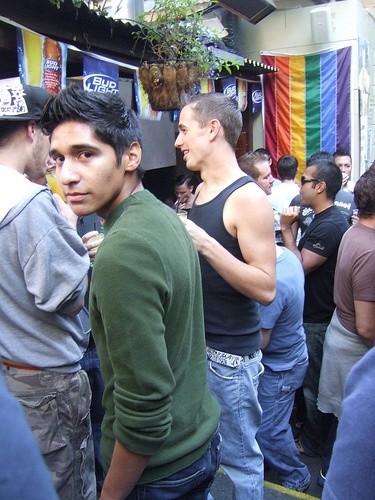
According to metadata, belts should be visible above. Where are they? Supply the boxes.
[207,347,255,369]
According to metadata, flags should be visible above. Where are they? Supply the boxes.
[15,26,263,121]
[259,45,353,186]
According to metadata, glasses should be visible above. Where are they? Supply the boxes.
[300,175,317,185]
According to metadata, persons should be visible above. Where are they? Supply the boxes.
[164,174,196,220]
[238,148,375,500]
[0,367,60,500]
[38,82,221,500]
[174,93,277,500]
[0,84,96,500]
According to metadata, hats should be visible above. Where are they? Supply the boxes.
[0,83,54,121]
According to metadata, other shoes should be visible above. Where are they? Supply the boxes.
[318,469,326,486]
[296,479,311,492]
